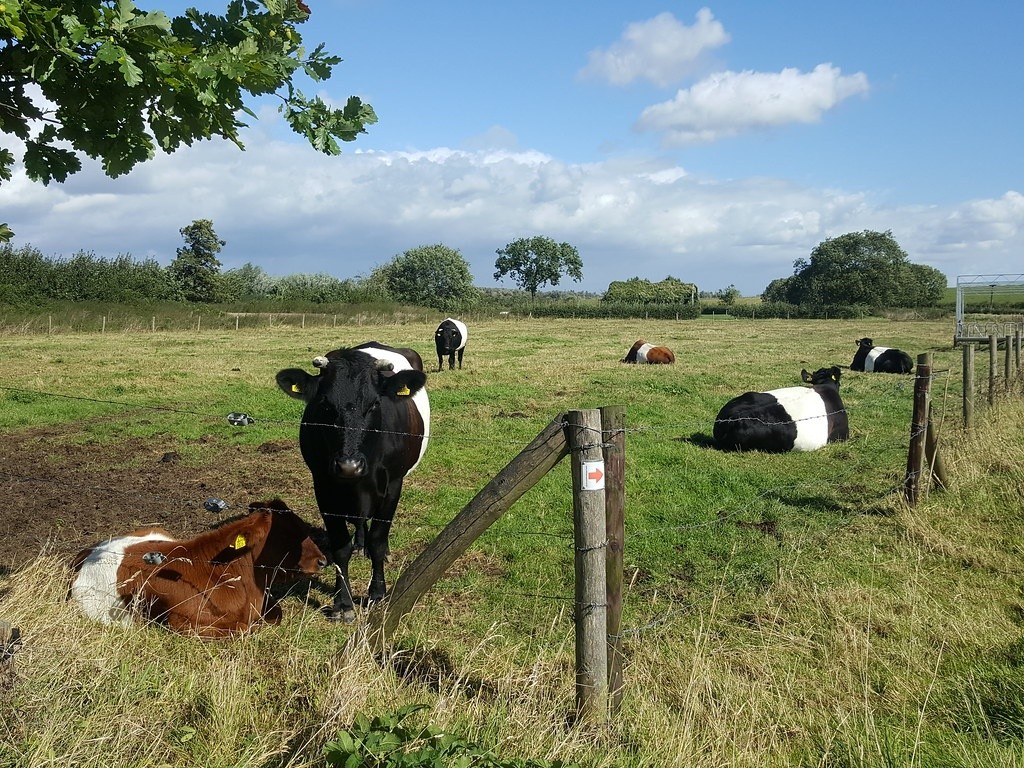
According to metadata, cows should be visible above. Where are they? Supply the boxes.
[435,317,468,370]
[71,499,326,639]
[625,339,675,364]
[713,366,849,453]
[275,340,431,622]
[837,337,913,374]
